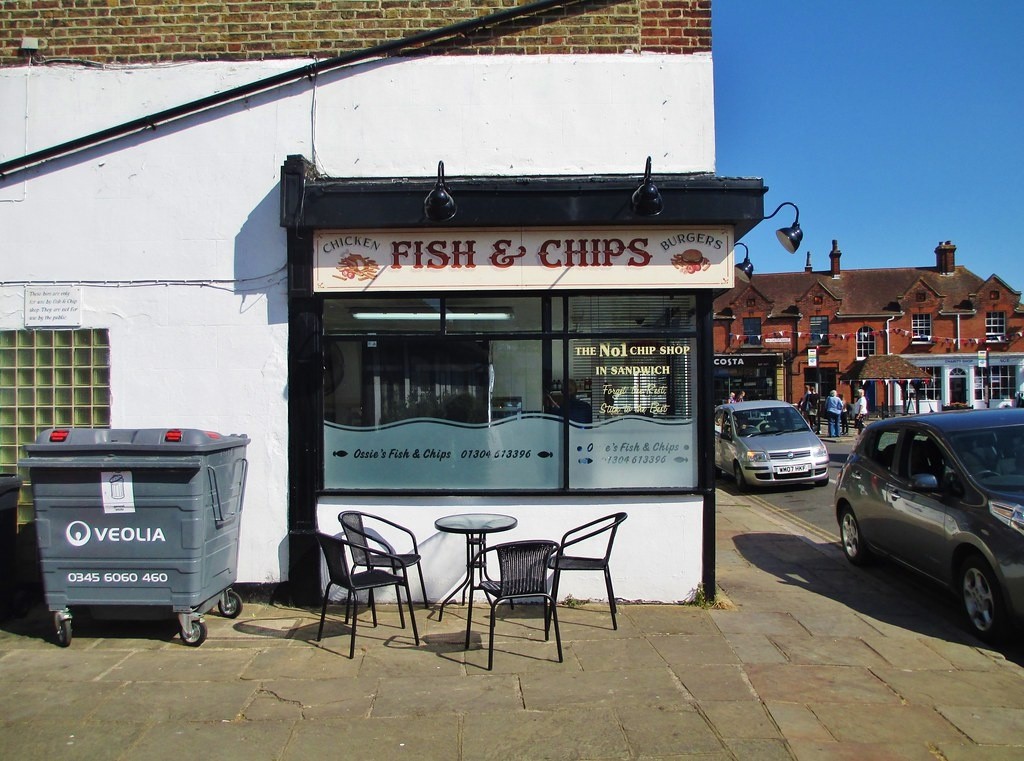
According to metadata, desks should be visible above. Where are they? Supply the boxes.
[432,513,518,623]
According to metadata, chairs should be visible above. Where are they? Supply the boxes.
[338,510,428,628]
[465,540,563,670]
[547,512,628,630]
[312,530,419,658]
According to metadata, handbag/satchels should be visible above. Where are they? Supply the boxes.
[809,409,818,415]
[802,395,809,412]
[837,399,842,410]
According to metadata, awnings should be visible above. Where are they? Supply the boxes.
[842,356,934,383]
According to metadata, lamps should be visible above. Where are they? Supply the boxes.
[627,156,668,219]
[763,202,804,255]
[424,162,457,222]
[732,241,756,281]
[348,307,515,321]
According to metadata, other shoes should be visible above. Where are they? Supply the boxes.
[835,435,840,437]
[815,432,820,434]
[840,432,848,435]
[827,435,832,438]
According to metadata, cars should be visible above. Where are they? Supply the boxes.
[833,408,1024,645]
[714,400,829,491]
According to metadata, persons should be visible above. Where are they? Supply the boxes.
[854,389,867,439]
[554,377,614,423]
[800,385,819,431]
[727,391,745,404]
[736,414,750,436]
[825,389,849,438]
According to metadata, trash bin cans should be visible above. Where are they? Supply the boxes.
[14,428,252,648]
[0,471,25,622]
